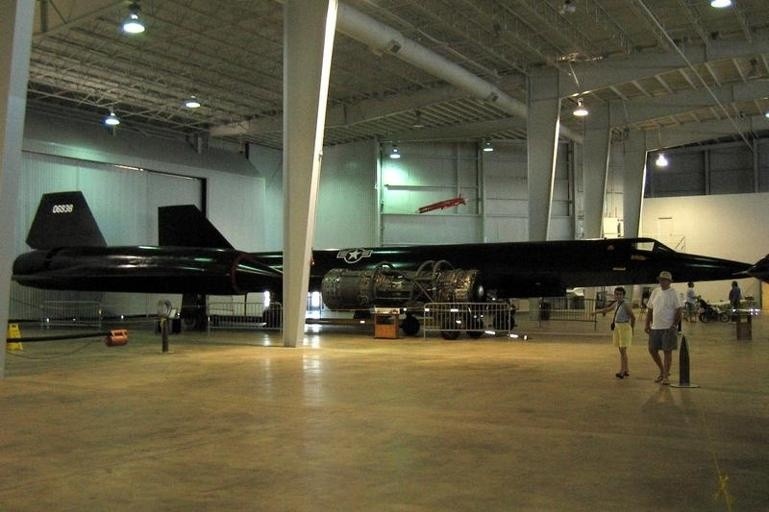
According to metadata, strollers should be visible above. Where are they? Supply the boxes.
[694,295,731,324]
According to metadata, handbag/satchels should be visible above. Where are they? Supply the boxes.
[610,322,615,330]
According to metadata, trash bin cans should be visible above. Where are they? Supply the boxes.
[574,296,585,309]
[374,313,400,339]
[735,314,752,340]
[538,302,551,321]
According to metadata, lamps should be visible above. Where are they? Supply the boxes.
[104,104,123,129]
[180,85,203,109]
[120,1,149,36]
[572,93,590,118]
[410,109,426,131]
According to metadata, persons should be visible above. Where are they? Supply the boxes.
[643,271,682,384]
[589,286,636,379]
[696,295,710,322]
[729,281,743,322]
[685,282,701,322]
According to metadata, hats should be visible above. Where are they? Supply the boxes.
[658,271,673,282]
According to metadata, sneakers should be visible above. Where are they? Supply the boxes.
[655,375,671,384]
[616,371,629,379]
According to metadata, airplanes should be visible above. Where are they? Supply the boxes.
[5,184,769,343]
[730,253,769,284]
